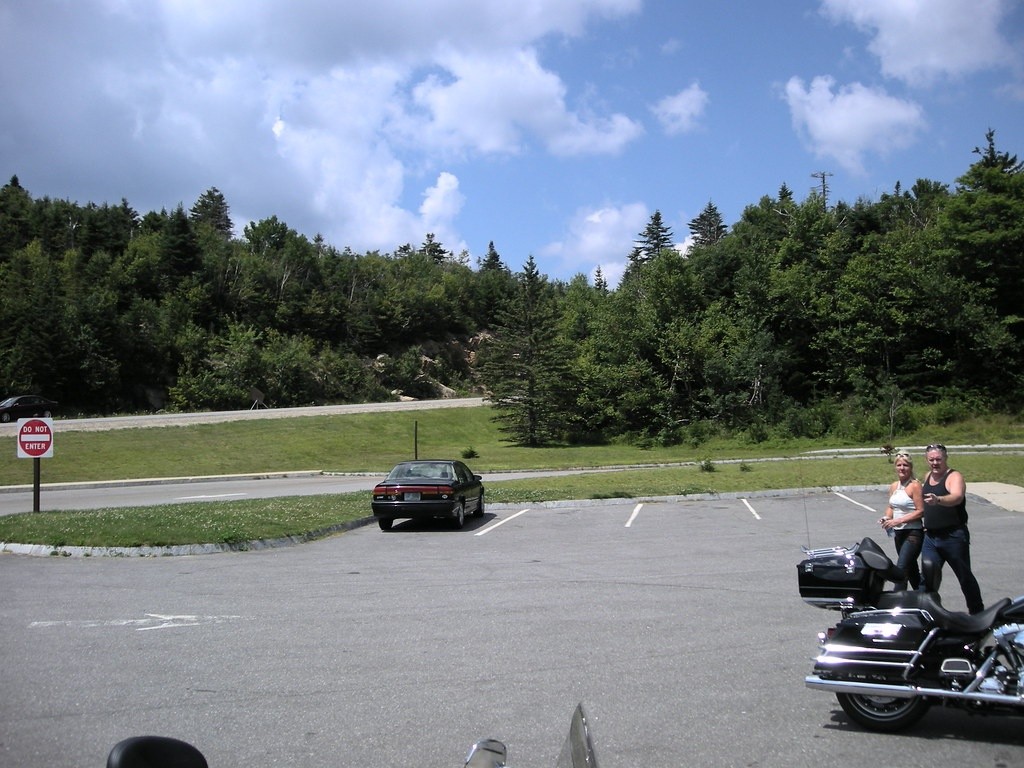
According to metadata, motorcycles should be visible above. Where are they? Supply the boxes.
[795,537,1024,732]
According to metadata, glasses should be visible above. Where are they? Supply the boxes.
[926,444,947,453]
[895,453,909,457]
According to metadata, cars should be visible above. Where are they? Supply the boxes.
[0,395,61,423]
[370,458,485,531]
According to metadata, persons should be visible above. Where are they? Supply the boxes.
[879,450,925,590]
[915,445,983,616]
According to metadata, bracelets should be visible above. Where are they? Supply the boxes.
[938,498,941,504]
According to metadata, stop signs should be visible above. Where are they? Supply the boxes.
[16,419,53,459]
